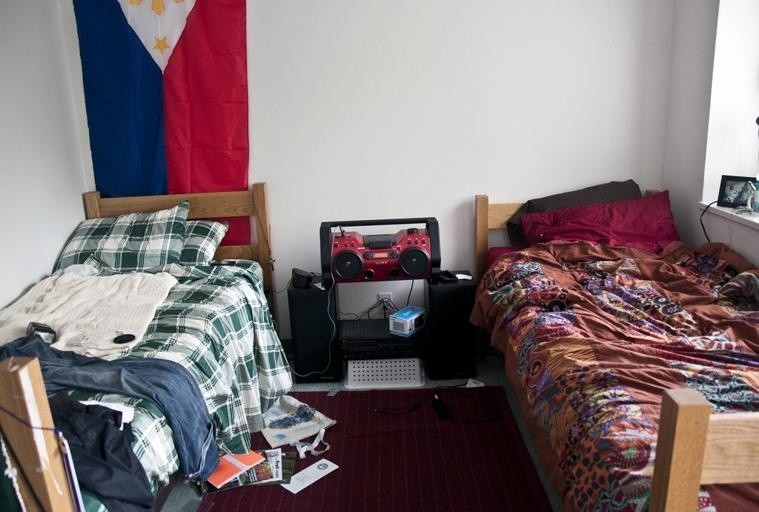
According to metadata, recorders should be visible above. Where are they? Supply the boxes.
[329,225,434,281]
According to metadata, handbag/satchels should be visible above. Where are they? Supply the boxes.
[262,394,336,459]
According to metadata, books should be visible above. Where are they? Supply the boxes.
[198,446,340,498]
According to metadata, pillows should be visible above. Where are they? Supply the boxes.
[54,199,231,269]
[506,176,678,262]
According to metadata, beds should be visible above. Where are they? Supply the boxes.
[472,190,759,512]
[0,184,275,510]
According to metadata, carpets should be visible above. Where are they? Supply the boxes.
[198,386,552,511]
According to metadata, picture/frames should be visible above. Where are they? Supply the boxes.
[716,175,757,207]
